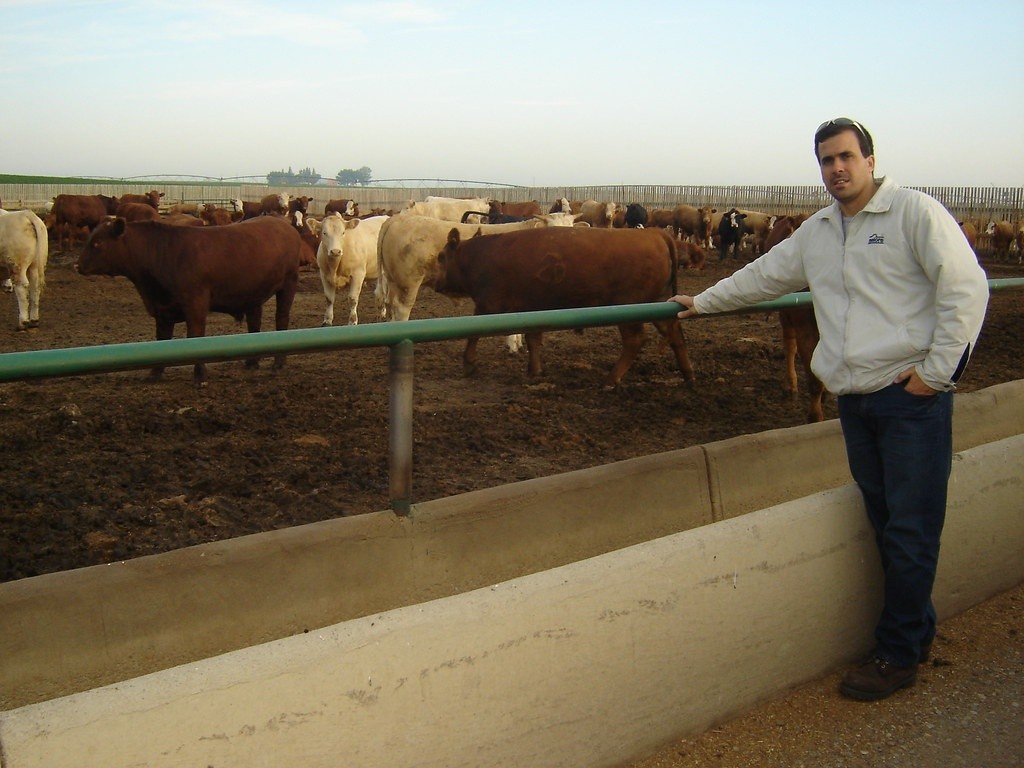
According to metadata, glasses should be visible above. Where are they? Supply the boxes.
[815,117,869,141]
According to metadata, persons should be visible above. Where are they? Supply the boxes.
[666,117,990,702]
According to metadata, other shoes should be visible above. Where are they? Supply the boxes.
[839,650,918,701]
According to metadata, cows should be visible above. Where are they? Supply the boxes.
[958,220,1024,265]
[0,191,825,421]
